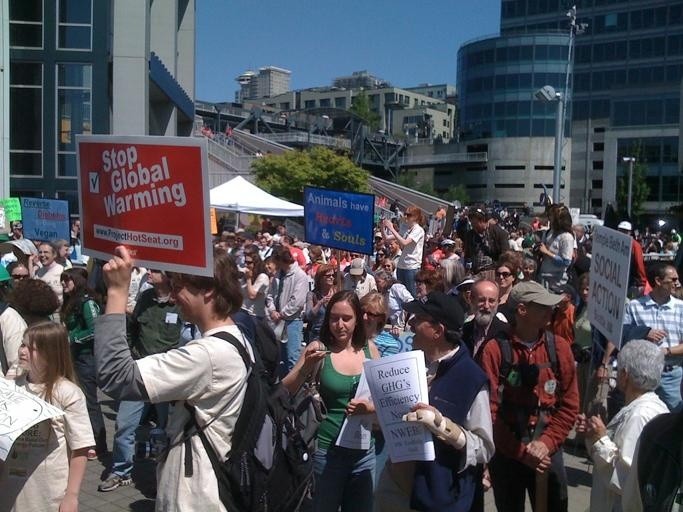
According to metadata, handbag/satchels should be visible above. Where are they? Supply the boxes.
[287,344,329,458]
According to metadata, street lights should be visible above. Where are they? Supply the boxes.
[535,83,566,208]
[622,156,635,220]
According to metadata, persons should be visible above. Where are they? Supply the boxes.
[549,284,577,346]
[283,290,380,512]
[0,323,96,511]
[389,238,402,263]
[305,264,337,344]
[6,261,29,282]
[620,377,683,512]
[581,324,616,465]
[539,202,575,286]
[344,258,376,299]
[70,220,81,246]
[256,150,262,157]
[390,199,400,216]
[98,268,187,492]
[10,220,23,240]
[478,281,580,512]
[383,205,426,298]
[374,290,496,511]
[375,248,388,272]
[233,252,269,323]
[374,272,414,340]
[264,256,281,306]
[35,241,64,323]
[93,246,315,512]
[416,200,545,289]
[308,245,373,263]
[575,338,665,512]
[623,260,683,412]
[576,217,683,272]
[374,232,387,250]
[267,248,309,380]
[58,269,108,461]
[360,291,398,363]
[0,277,60,375]
[573,273,593,349]
[213,216,303,252]
[54,240,73,270]
[383,256,397,289]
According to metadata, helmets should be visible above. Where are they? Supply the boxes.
[521,235,536,249]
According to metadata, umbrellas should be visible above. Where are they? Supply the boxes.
[210,176,305,232]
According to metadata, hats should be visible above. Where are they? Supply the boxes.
[374,232,383,239]
[518,220,532,233]
[507,281,565,306]
[12,221,23,228]
[440,239,456,246]
[402,290,465,331]
[426,238,440,244]
[469,207,485,216]
[350,258,365,275]
[456,278,476,291]
[618,221,632,231]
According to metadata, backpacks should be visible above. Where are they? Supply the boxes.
[185,326,315,512]
[495,329,561,442]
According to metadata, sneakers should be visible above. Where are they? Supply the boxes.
[149,452,156,461]
[88,443,107,459]
[99,472,133,492]
[481,473,492,491]
[134,443,146,462]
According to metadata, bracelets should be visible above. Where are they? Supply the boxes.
[666,347,671,358]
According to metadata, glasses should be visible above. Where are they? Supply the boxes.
[244,252,250,255]
[244,260,254,265]
[415,281,426,285]
[9,274,30,279]
[384,264,391,268]
[146,268,161,273]
[350,254,360,259]
[323,274,336,278]
[495,271,512,277]
[376,253,386,257]
[665,276,679,282]
[404,211,414,218]
[60,277,73,283]
